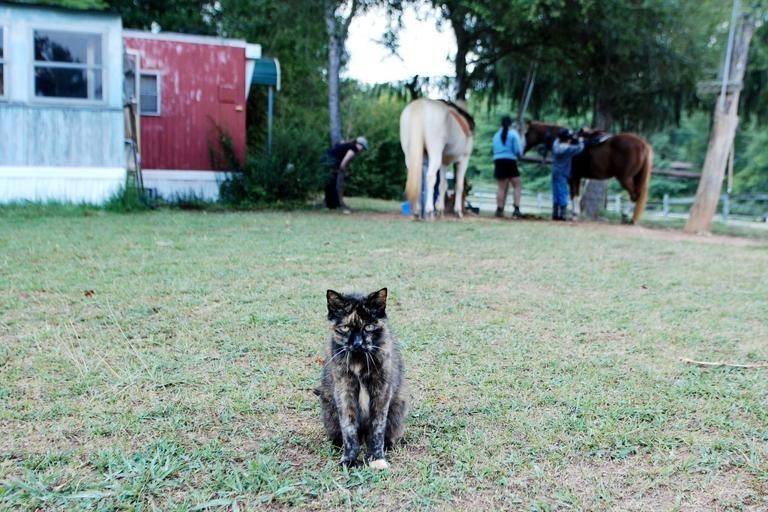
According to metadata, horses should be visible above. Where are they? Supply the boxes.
[523,119,653,225]
[399,96,475,223]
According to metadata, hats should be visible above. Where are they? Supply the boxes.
[356,137,369,151]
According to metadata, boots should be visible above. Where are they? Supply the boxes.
[552,205,573,221]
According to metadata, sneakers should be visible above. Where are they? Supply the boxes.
[495,206,527,219]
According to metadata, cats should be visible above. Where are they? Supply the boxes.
[313,286,408,471]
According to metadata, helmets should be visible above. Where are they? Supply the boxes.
[557,129,572,140]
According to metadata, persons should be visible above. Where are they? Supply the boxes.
[491,115,529,218]
[320,135,369,215]
[551,127,585,221]
